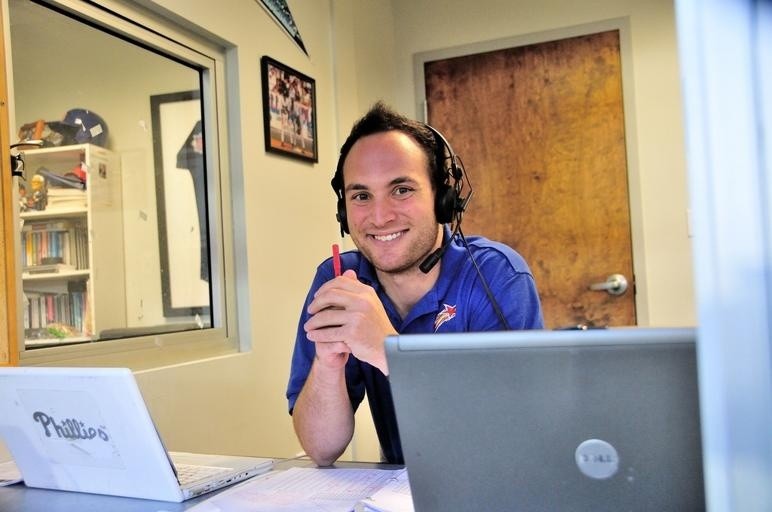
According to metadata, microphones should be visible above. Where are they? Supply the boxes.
[419,216,464,274]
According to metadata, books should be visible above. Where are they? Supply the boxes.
[18,165,96,343]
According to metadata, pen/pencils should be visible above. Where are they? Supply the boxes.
[332,245,341,277]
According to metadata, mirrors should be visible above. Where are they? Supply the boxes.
[2,0,239,371]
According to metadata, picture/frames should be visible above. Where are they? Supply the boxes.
[151,90,210,317]
[262,56,317,163]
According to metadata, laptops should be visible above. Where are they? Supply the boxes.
[1,364,274,504]
[384,326,706,512]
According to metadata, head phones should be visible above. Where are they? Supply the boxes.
[330,125,470,237]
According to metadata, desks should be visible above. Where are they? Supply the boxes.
[0,456,405,512]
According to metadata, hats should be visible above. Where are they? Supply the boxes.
[44,108,108,146]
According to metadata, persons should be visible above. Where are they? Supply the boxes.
[288,101,544,467]
[266,64,314,156]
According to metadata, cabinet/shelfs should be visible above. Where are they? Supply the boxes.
[17,143,127,348]
